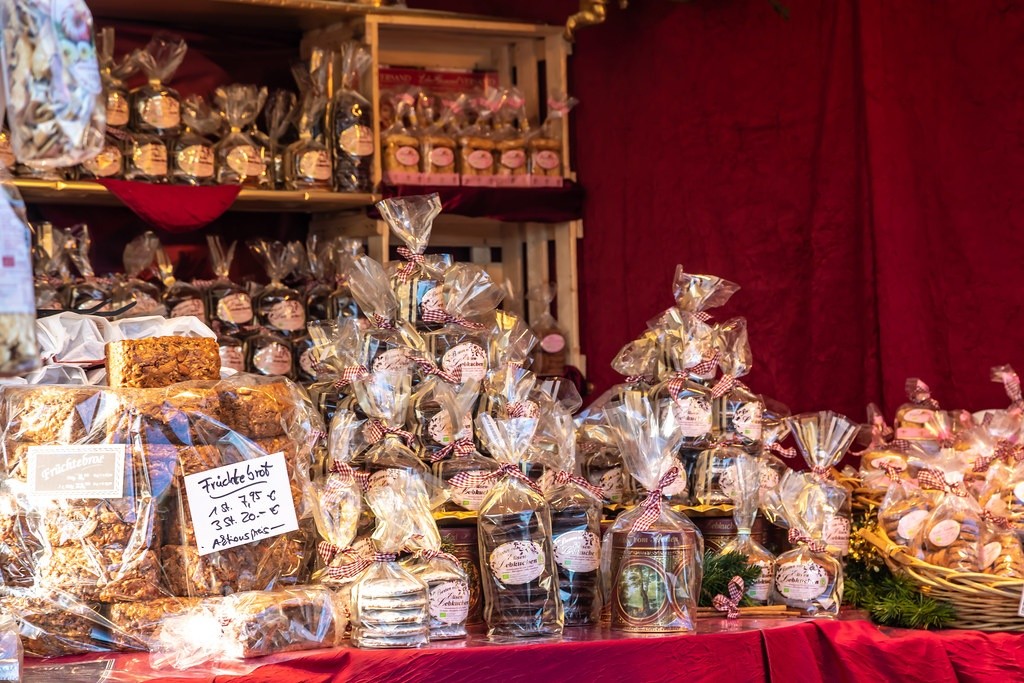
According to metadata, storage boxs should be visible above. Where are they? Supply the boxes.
[300,15,575,188]
[315,212,589,380]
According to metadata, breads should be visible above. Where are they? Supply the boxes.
[0,85,1024,660]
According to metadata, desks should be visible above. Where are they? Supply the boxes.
[20,611,1024,683]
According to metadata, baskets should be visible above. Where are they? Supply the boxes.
[858,492,1024,632]
[847,486,882,529]
